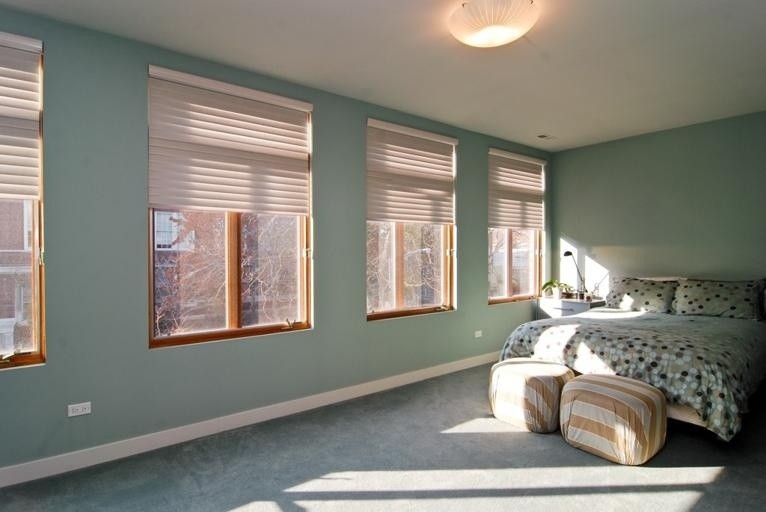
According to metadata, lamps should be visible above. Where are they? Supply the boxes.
[446,0,545,48]
[564,251,588,292]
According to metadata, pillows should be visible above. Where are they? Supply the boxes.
[605,271,766,321]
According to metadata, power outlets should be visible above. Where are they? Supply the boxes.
[68,401,91,417]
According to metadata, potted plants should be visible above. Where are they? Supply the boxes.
[541,278,575,299]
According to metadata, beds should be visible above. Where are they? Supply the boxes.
[503,304,766,442]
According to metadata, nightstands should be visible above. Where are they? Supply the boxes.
[537,297,605,320]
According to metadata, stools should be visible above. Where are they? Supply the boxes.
[488,357,667,466]
